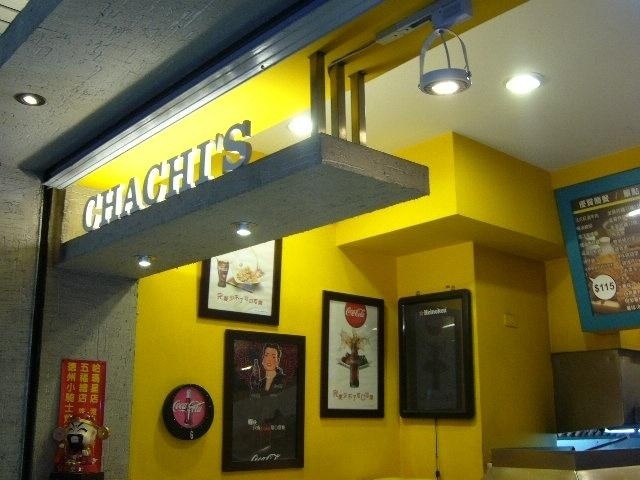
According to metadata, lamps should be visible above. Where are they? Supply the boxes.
[418,27,471,96]
[137,255,153,267]
[235,222,253,236]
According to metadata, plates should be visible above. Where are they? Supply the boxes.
[233,271,269,286]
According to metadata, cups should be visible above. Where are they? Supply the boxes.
[217,258,229,287]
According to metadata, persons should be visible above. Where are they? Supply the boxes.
[249,344,287,399]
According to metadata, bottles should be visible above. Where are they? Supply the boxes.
[250,360,260,395]
[594,236,621,297]
[349,343,361,390]
[183,389,194,426]
[260,404,273,452]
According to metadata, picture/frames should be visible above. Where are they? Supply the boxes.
[553,167,640,332]
[222,329,305,472]
[198,238,283,325]
[397,289,476,419]
[321,290,384,418]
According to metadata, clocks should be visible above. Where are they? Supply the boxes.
[163,383,215,440]
